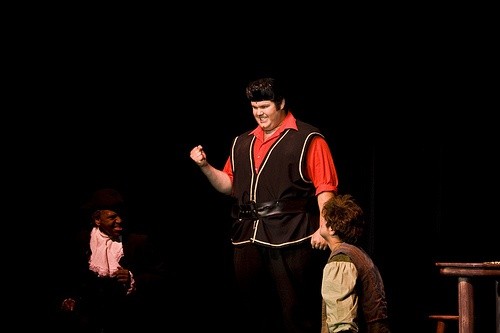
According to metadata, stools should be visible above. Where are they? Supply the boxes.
[430,314,460,333]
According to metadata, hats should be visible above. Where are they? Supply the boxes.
[82,188,124,212]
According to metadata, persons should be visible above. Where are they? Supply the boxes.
[317,193,394,333]
[190,78,341,333]
[59,188,172,333]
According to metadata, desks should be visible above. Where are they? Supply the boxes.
[435,261,496,333]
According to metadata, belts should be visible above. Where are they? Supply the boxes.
[231,196,316,219]
